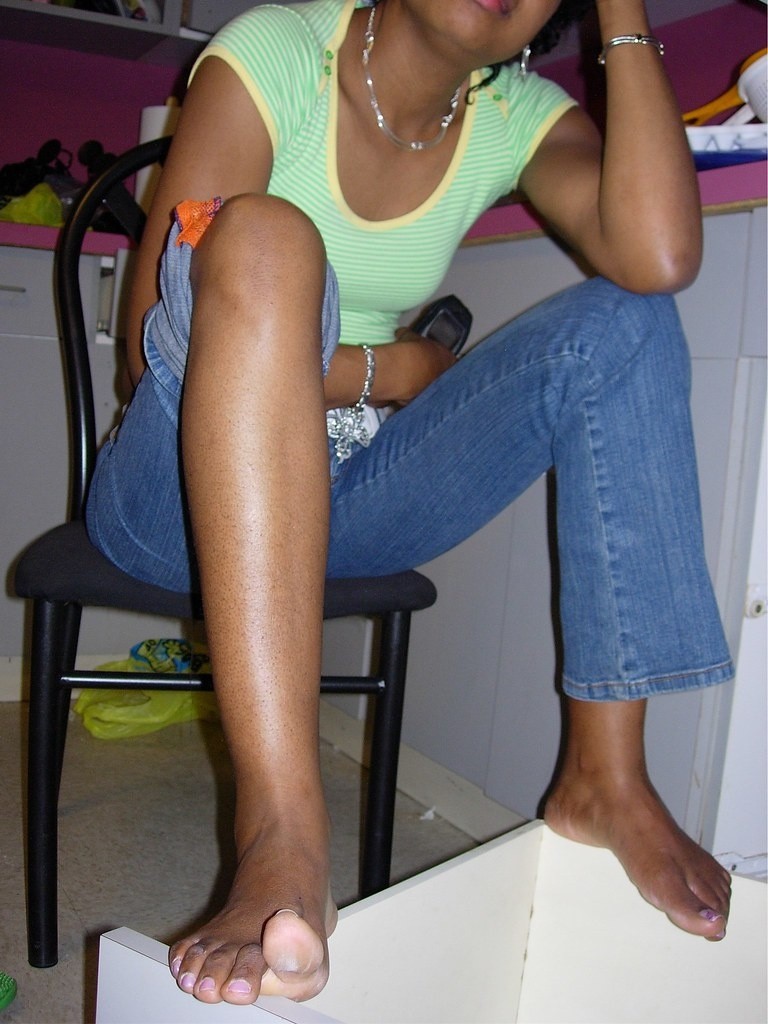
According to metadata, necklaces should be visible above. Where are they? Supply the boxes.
[362,7,461,150]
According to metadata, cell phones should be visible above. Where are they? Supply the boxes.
[410,295,474,356]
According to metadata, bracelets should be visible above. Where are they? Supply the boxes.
[357,345,375,406]
[599,34,664,64]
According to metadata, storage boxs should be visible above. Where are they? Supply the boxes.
[94,820,768,1024]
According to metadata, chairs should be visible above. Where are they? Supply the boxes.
[7,134,435,966]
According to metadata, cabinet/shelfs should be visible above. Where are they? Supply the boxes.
[0,0,274,70]
[0,250,205,699]
[320,206,765,879]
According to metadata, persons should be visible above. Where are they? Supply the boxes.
[89,0,733,1006]
